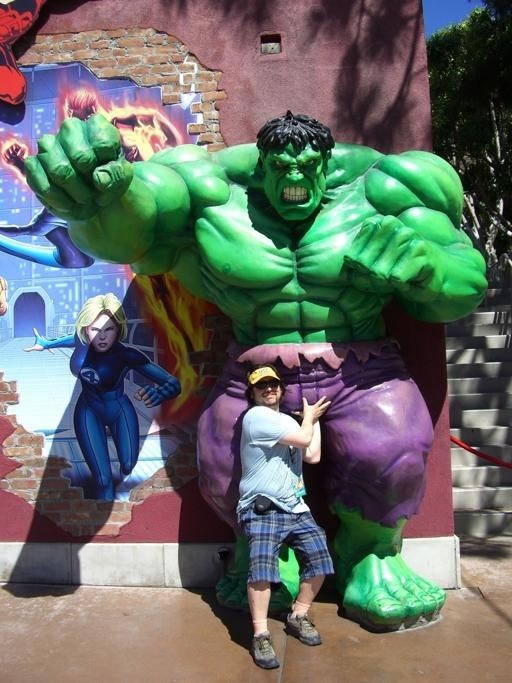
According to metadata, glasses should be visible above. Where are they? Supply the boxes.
[254,380,279,389]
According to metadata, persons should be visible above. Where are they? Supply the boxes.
[235,361,333,668]
[21,104,489,629]
[22,293,182,500]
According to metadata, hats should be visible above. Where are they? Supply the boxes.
[248,366,281,385]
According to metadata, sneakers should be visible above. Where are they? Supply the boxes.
[249,634,280,669]
[285,612,322,646]
